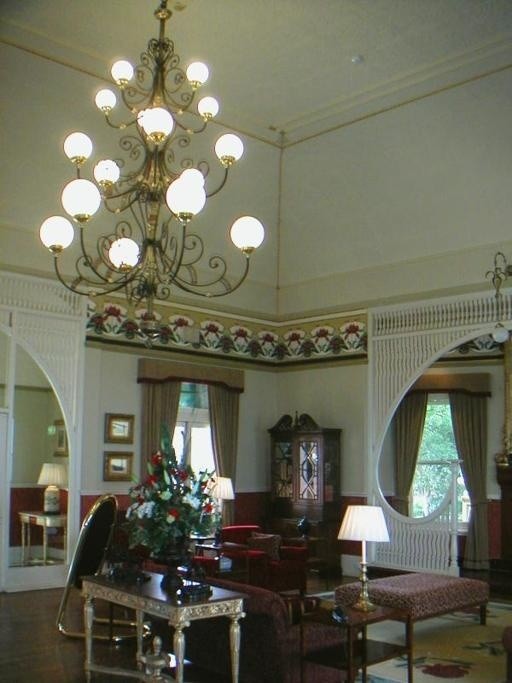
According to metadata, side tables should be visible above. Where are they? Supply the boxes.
[193,542,250,584]
[298,601,415,683]
[18,510,67,567]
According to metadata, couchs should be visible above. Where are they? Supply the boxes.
[143,554,357,682]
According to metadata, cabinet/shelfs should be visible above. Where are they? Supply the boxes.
[268,427,343,580]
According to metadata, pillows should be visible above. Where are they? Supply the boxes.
[246,531,283,563]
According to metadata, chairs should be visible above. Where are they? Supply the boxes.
[216,524,309,595]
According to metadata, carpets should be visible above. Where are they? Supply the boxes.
[307,589,512,683]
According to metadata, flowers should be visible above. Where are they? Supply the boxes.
[124,418,222,559]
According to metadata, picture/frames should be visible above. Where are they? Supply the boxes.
[53,419,68,457]
[103,413,134,444]
[102,452,134,482]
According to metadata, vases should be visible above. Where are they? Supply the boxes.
[153,543,185,592]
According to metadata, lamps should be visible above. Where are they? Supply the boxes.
[338,505,390,612]
[36,462,68,515]
[203,477,235,529]
[484,251,511,344]
[39,1,265,349]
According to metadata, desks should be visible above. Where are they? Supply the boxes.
[77,567,249,683]
[185,531,217,556]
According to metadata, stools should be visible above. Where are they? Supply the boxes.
[334,572,490,683]
